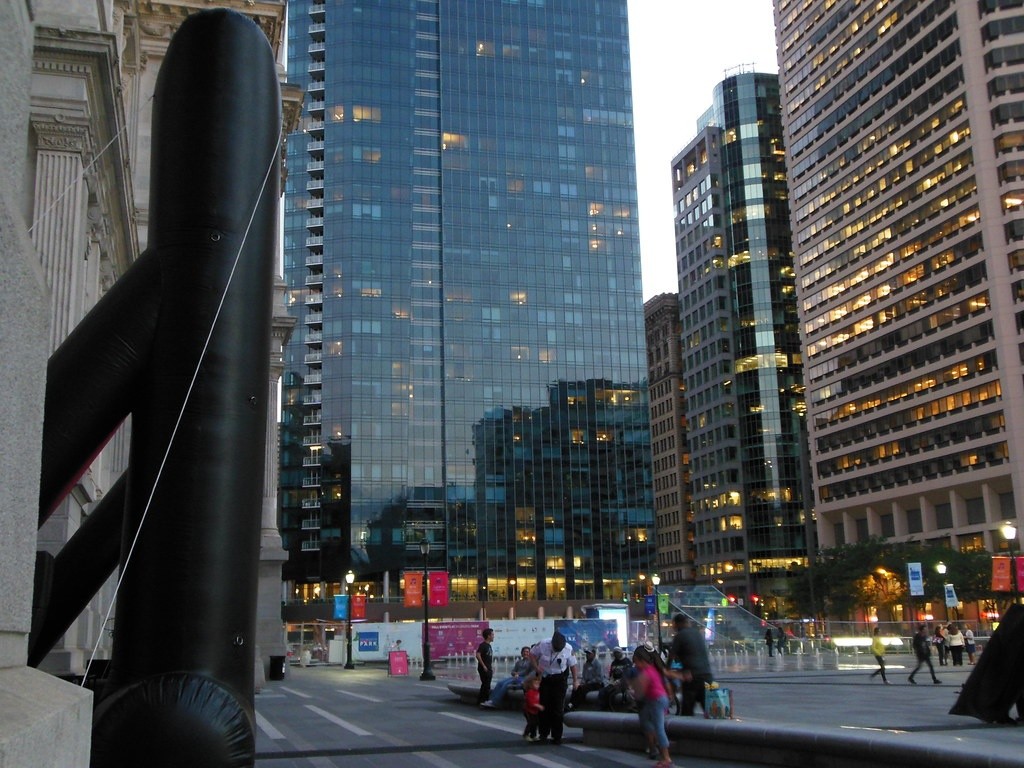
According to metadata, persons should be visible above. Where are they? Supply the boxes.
[480,614,714,768]
[476,628,495,709]
[869,627,892,684]
[776,627,786,657]
[933,622,977,665]
[765,628,774,658]
[907,625,942,684]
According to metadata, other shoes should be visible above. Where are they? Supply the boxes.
[480,700,496,707]
[532,737,540,741]
[524,735,532,742]
[934,680,942,684]
[657,760,672,768]
[908,678,917,685]
[884,681,891,685]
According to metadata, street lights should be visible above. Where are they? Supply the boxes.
[1002,521,1020,604]
[420,538,436,681]
[936,560,950,621]
[344,569,356,669]
[651,573,664,652]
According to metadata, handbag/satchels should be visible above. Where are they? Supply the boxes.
[932,636,944,645]
[704,687,734,720]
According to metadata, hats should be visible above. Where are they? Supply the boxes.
[584,648,595,656]
[613,646,623,654]
